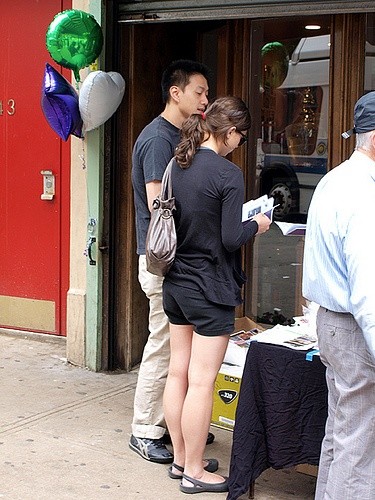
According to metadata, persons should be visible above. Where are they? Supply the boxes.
[127,66,216,464]
[156,96,270,495]
[301,91,374,500]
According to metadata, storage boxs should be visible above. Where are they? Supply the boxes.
[211,318,266,435]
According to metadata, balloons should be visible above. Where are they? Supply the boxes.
[78,71,125,131]
[41,62,84,141]
[44,9,104,82]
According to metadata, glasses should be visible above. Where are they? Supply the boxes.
[236,130,248,146]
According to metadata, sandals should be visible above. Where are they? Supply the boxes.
[169,459,230,494]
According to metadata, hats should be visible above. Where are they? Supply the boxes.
[341,91,375,139]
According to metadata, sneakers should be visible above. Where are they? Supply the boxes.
[129,428,215,464]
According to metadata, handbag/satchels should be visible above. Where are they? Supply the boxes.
[146,197,177,278]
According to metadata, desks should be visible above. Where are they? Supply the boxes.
[226,315,332,500]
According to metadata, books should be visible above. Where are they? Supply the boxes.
[242,193,280,226]
[274,221,306,236]
